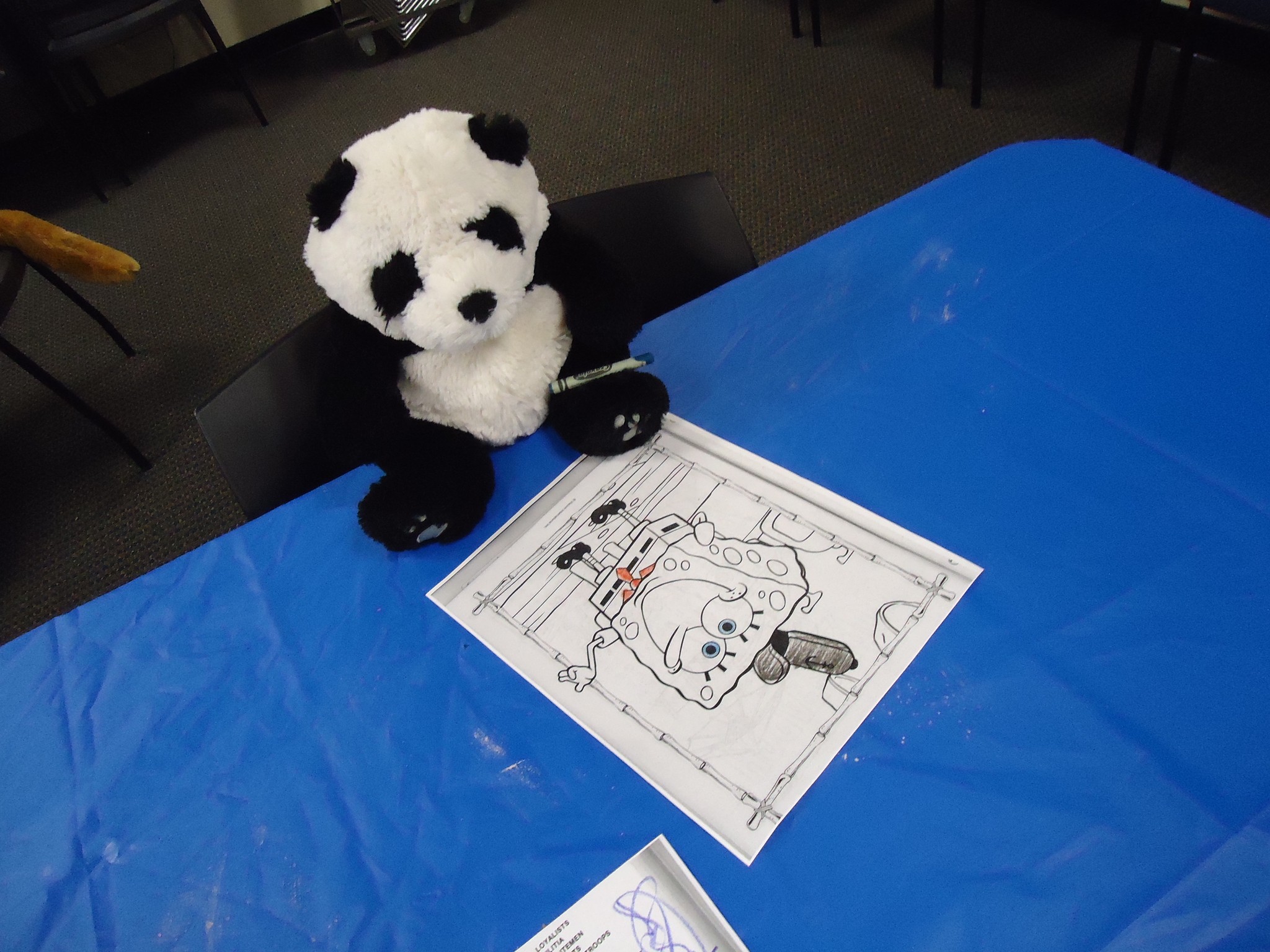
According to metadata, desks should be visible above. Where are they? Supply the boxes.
[1,143,1269,952]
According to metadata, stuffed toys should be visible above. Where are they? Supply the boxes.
[298,105,668,550]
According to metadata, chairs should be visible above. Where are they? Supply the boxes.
[27,0,269,188]
[193,172,762,522]
[1,245,158,471]
